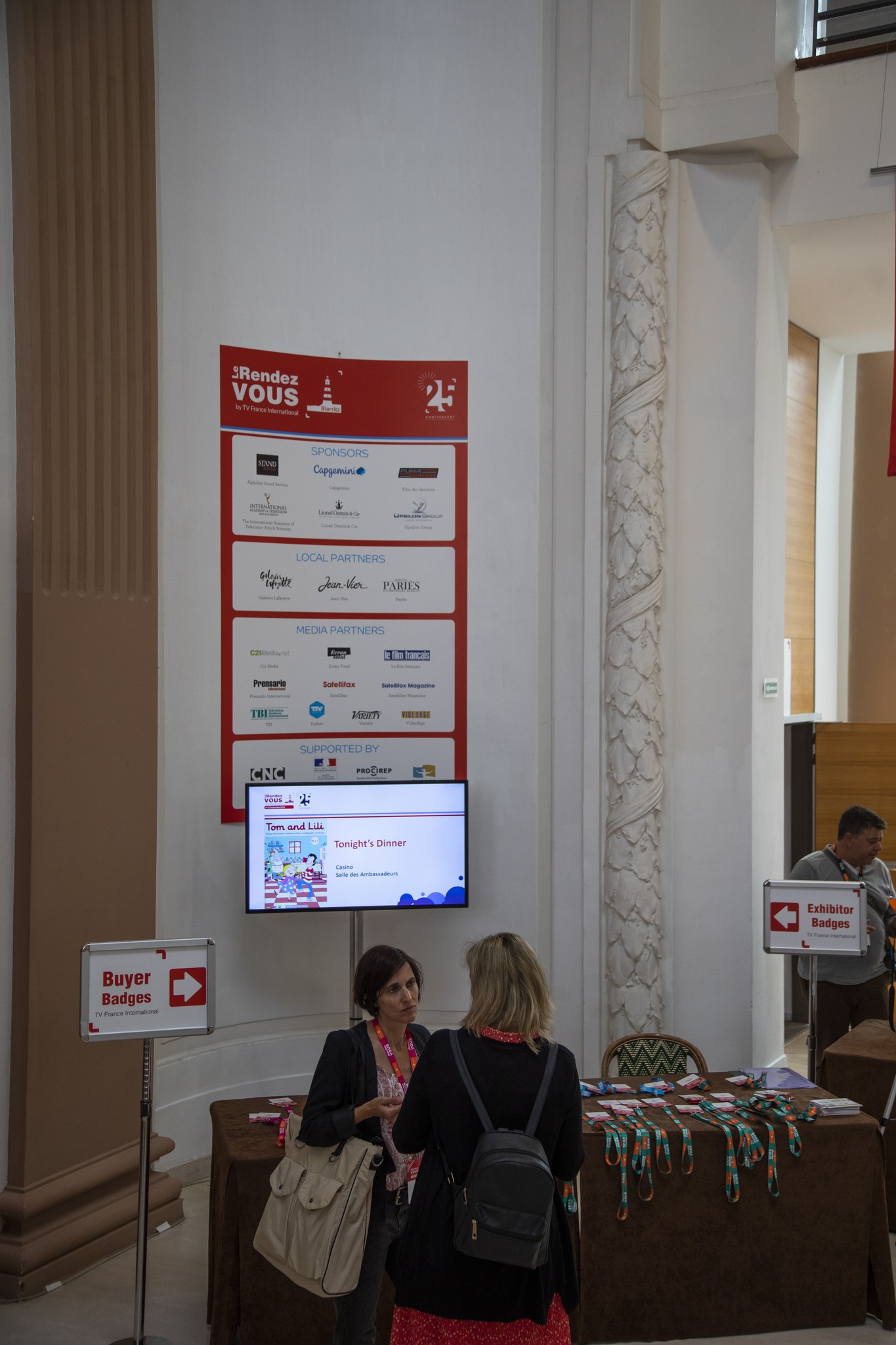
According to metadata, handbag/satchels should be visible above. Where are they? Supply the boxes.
[252,1112,384,1299]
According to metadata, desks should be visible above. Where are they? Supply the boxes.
[819,1018,896,1234]
[207,1066,896,1345]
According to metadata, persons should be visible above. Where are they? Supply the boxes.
[786,804,896,1093]
[389,931,587,1345]
[295,944,432,1344]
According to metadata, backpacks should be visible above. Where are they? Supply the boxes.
[436,1029,559,1270]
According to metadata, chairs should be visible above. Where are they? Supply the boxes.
[602,1033,708,1078]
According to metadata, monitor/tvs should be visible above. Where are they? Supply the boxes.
[244,780,470,914]
